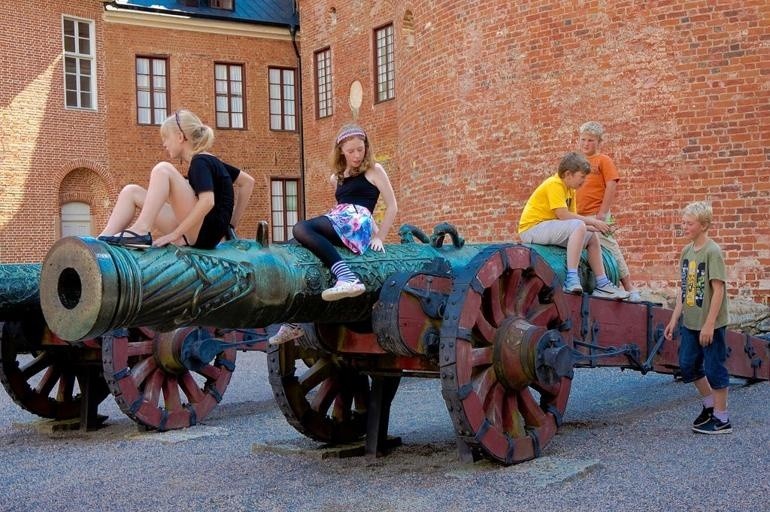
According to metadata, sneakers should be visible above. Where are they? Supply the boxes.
[268,323,304,345]
[591,280,641,302]
[563,276,584,295]
[690,405,734,434]
[321,278,366,302]
[98,228,153,247]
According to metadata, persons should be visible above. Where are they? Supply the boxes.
[267,123,399,348]
[519,151,632,301]
[96,109,253,249]
[576,119,642,305]
[662,201,734,434]
